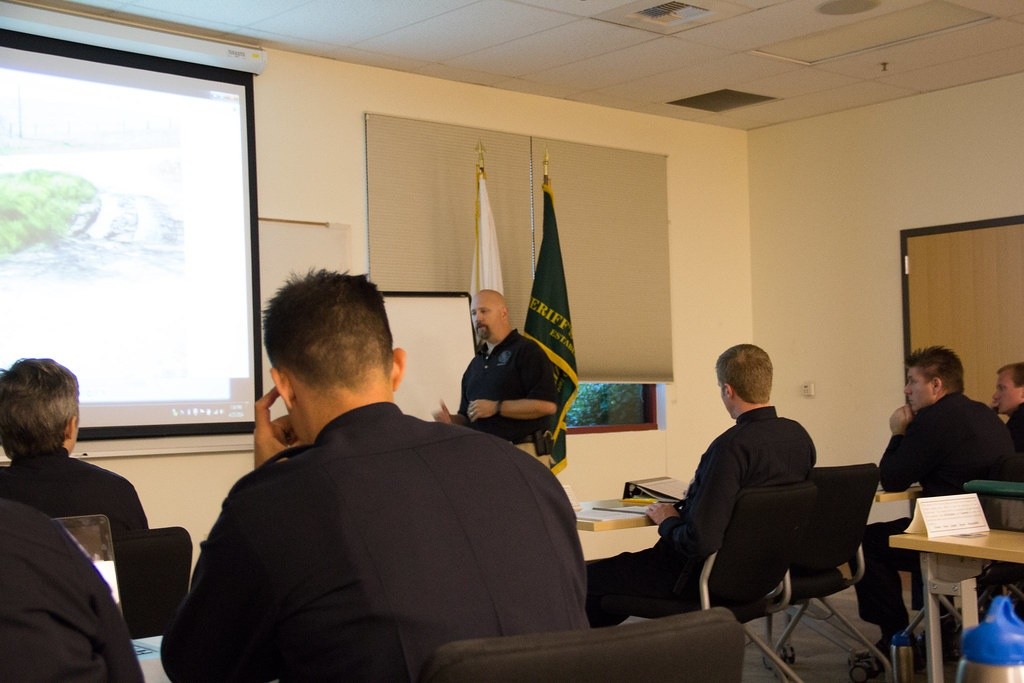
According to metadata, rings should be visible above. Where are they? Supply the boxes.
[470,412,473,415]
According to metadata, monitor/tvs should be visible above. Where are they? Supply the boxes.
[53,515,124,621]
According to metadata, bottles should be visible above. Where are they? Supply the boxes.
[955,596,1024,683]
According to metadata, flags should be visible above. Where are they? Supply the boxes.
[523,181,579,474]
[470,174,505,295]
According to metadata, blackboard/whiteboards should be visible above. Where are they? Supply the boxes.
[377,291,480,423]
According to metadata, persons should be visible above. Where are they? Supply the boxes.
[587,344,816,629]
[0,501,146,683]
[159,270,590,683]
[988,362,1024,453]
[431,290,560,469]
[847,347,1018,669]
[0,358,150,532]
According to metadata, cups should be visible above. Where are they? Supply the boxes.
[890,631,914,683]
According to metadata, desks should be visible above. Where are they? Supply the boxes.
[131,636,281,683]
[889,527,1024,683]
[569,493,876,531]
[876,485,923,517]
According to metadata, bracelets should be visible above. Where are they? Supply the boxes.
[496,399,504,415]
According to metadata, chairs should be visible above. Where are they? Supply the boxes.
[109,464,1024,683]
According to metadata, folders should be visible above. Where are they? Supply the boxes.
[622,475,690,503]
